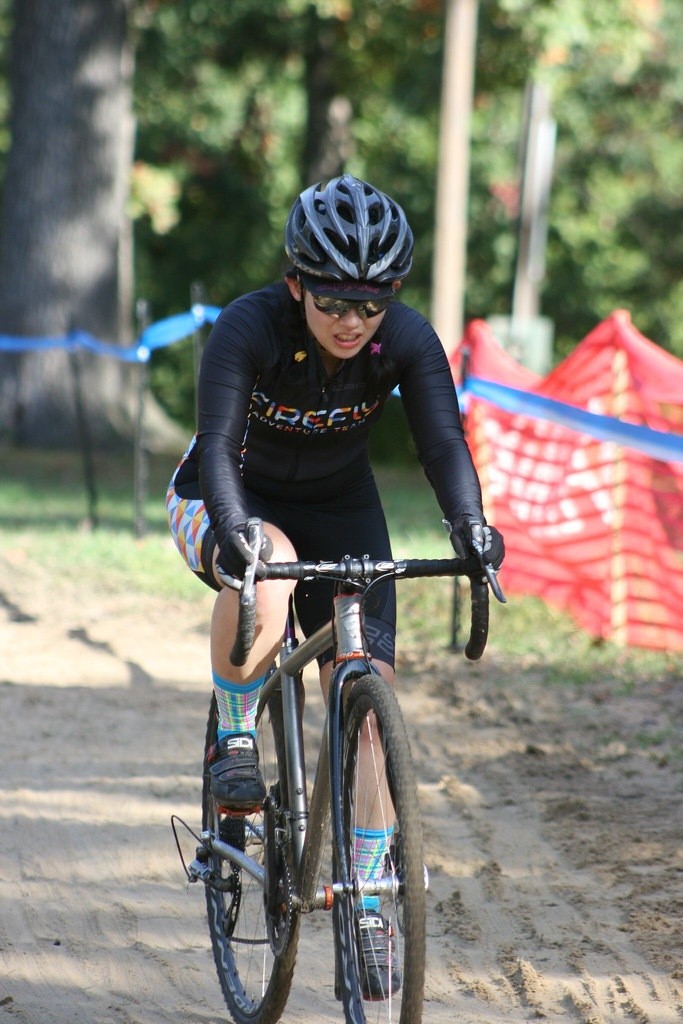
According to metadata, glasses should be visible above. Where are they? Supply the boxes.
[311,293,390,320]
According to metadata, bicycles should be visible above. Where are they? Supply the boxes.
[169,513,507,1024]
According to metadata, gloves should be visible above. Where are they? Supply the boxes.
[215,523,274,593]
[449,514,505,585]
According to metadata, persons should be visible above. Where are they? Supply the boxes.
[165,174,505,1000]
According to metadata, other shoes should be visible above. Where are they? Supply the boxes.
[206,733,268,818]
[351,906,401,1002]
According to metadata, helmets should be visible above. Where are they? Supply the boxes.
[284,173,415,302]
